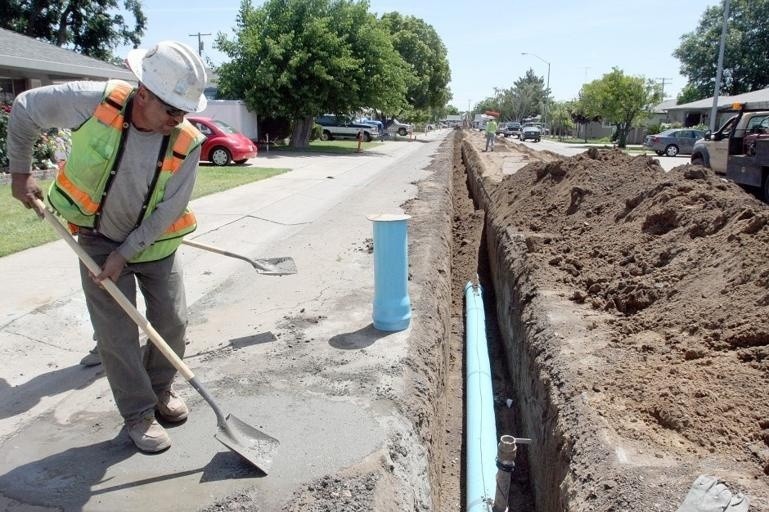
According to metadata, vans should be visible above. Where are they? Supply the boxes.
[478,119,489,131]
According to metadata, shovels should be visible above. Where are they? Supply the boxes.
[181,239,297,275]
[31,195,280,475]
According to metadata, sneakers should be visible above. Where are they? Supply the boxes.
[80,344,101,365]
[155,387,189,421]
[126,416,172,452]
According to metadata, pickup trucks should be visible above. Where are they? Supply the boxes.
[503,121,522,138]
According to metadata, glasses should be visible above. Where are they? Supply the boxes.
[145,88,183,117]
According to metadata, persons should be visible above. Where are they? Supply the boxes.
[482,119,497,152]
[7,39,209,453]
[406,122,413,142]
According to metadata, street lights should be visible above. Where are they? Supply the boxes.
[521,51,551,137]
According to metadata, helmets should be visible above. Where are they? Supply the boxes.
[127,40,207,113]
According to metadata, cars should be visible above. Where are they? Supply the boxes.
[519,126,541,141]
[436,122,447,128]
[643,128,705,157]
[187,115,258,166]
[412,123,436,131]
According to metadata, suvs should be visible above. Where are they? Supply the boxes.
[352,112,384,139]
[386,118,413,136]
[498,122,508,133]
[315,113,379,142]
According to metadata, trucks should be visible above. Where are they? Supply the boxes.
[691,111,769,204]
